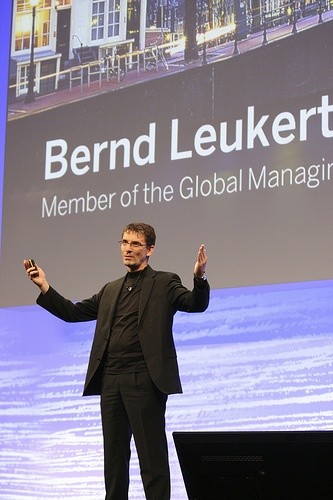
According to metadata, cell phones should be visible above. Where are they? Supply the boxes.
[28,259,39,276]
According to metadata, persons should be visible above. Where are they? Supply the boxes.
[24,223,211,500]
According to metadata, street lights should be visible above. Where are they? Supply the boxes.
[26,0,41,104]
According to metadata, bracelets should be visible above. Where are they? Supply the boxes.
[194,271,207,280]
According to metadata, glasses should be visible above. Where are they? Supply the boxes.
[118,241,150,247]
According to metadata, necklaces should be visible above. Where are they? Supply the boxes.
[126,271,141,291]
